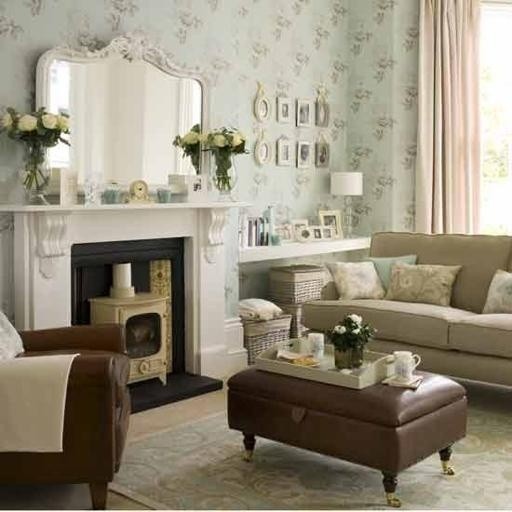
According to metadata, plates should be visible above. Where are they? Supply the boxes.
[287,359,320,367]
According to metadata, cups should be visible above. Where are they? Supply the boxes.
[105,190,120,204]
[157,190,172,203]
[393,350,422,383]
[81,168,104,208]
[308,332,326,362]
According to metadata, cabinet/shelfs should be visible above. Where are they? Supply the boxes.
[87,292,171,387]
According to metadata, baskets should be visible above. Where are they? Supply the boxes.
[274,303,314,339]
[242,313,292,365]
[266,263,327,304]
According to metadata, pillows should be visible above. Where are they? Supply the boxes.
[364,254,417,294]
[382,261,462,306]
[323,261,386,301]
[482,268,512,314]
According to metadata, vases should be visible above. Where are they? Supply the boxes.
[211,150,238,201]
[19,140,51,205]
[194,173,201,191]
[334,348,364,368]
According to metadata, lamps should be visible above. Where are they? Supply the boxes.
[330,173,362,239]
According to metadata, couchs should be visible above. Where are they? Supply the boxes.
[302,231,512,394]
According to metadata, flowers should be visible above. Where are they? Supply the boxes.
[1,106,71,190]
[173,122,200,191]
[197,126,250,190]
[325,310,379,348]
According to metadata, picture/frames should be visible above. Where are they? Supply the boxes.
[274,211,341,243]
[254,82,331,169]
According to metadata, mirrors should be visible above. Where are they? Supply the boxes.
[35,30,209,206]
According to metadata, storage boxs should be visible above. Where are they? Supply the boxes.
[243,264,324,365]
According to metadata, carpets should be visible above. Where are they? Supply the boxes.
[107,392,511,510]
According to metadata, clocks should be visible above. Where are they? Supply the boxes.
[130,179,149,203]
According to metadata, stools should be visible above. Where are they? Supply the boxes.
[228,367,468,507]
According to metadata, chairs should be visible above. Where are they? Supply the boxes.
[0,309,130,510]
